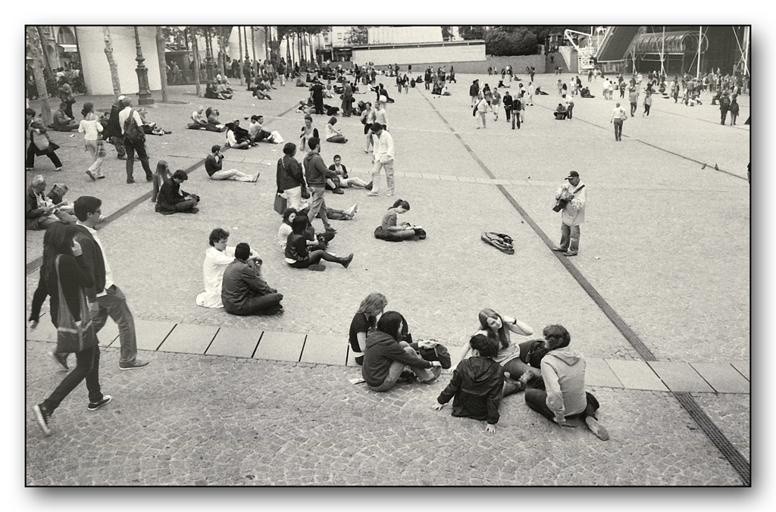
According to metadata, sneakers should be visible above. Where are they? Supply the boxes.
[31,403,52,437]
[118,358,152,371]
[552,247,568,252]
[252,170,260,182]
[87,394,112,411]
[365,181,373,190]
[85,170,96,181]
[423,366,442,385]
[585,415,609,441]
[367,190,379,196]
[49,350,71,372]
[563,250,578,256]
[345,203,358,220]
[333,187,344,194]
[326,227,338,234]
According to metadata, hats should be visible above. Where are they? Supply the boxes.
[564,170,579,180]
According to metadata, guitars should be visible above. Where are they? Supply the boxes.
[552,184,586,212]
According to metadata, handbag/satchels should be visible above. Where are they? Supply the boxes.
[66,95,76,103]
[55,287,101,354]
[33,131,50,151]
[299,136,305,151]
[473,106,478,116]
[273,191,288,215]
[420,340,452,369]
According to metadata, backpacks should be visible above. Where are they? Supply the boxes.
[122,109,146,148]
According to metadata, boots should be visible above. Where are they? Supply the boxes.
[308,263,326,272]
[336,253,354,269]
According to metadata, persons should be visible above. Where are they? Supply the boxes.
[203,228,283,316]
[190,101,419,271]
[26,61,153,183]
[166,62,455,116]
[348,293,610,441]
[27,175,150,438]
[470,65,535,130]
[553,60,748,140]
[152,160,200,214]
[553,171,586,256]
